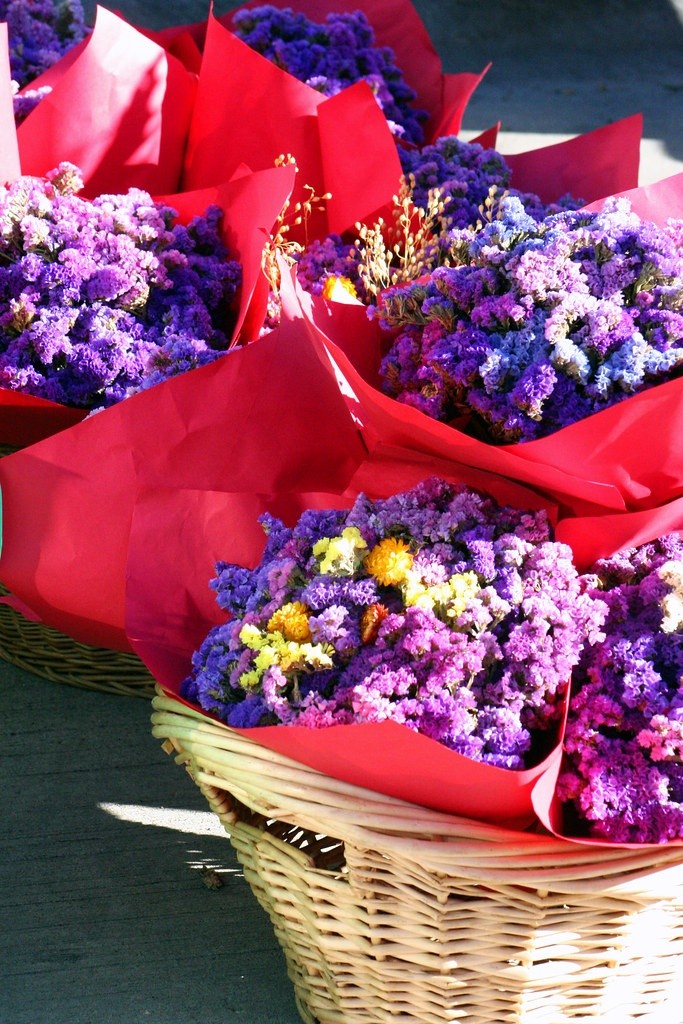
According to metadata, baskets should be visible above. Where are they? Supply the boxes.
[0,588,155,699]
[146,685,683,1024]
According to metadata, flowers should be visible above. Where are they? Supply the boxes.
[232,4,428,153]
[0,0,89,126]
[182,472,683,845]
[0,160,252,420]
[259,137,683,448]
